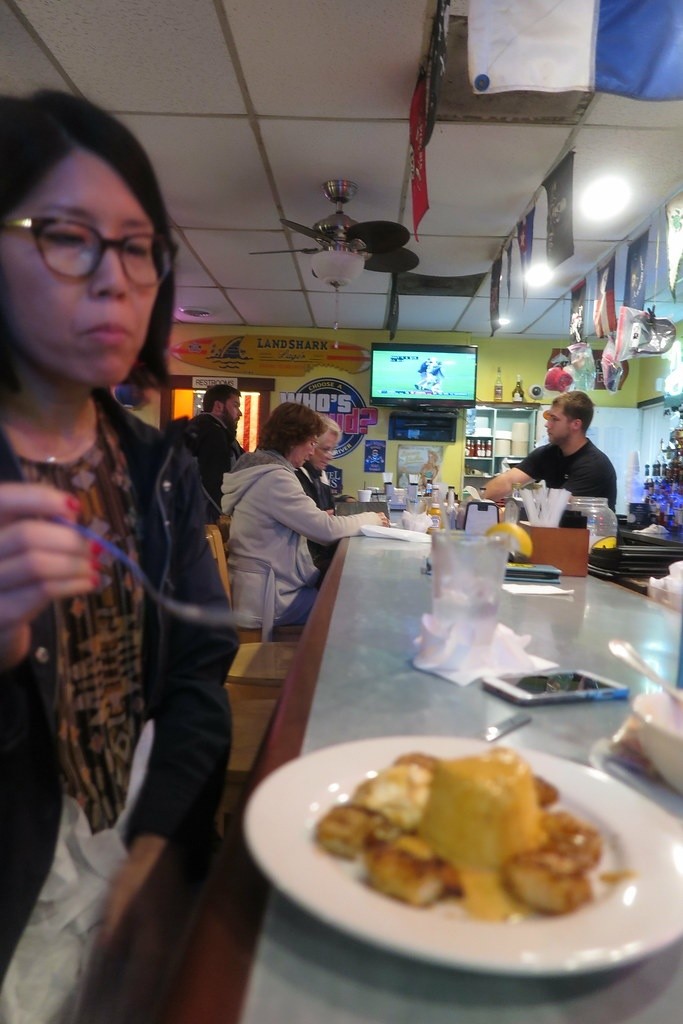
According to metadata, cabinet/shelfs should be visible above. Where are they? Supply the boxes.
[461,406,539,492]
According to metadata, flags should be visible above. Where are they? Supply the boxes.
[384,277,398,340]
[408,73,429,244]
[542,150,574,273]
[568,277,587,341]
[623,230,650,310]
[594,252,617,338]
[489,254,502,339]
[468,1,682,98]
[419,1,448,151]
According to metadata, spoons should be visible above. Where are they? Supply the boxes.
[65,519,254,628]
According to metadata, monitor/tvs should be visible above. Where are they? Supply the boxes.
[370,342,478,409]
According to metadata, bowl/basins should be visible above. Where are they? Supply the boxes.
[631,691,682,795]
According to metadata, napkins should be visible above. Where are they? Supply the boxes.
[411,613,560,689]
[361,510,434,543]
[501,584,574,595]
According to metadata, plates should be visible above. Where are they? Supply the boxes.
[241,733,683,975]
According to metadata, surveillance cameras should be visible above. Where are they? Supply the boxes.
[529,386,543,399]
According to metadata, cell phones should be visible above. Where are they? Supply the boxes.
[483,669,630,706]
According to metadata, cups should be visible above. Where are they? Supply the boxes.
[405,498,426,515]
[430,530,510,654]
[356,490,372,503]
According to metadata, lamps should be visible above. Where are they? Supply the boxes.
[310,252,364,350]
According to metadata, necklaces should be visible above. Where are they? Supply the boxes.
[48,457,56,463]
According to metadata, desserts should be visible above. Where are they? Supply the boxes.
[424,746,548,870]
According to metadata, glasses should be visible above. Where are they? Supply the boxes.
[308,439,319,448]
[2,218,178,286]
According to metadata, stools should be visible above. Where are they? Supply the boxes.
[203,517,300,835]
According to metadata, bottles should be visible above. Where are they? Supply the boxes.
[494,367,503,402]
[445,486,456,530]
[427,503,443,535]
[479,487,486,500]
[568,496,617,552]
[512,374,523,402]
[465,439,492,458]
[424,480,433,505]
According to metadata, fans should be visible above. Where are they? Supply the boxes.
[247,180,419,273]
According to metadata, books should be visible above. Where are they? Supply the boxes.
[424,558,563,583]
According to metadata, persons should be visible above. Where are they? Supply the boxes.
[223,402,390,628]
[299,416,342,589]
[182,387,243,506]
[414,357,445,392]
[482,391,617,527]
[0,89,240,1024]
[420,449,439,489]
